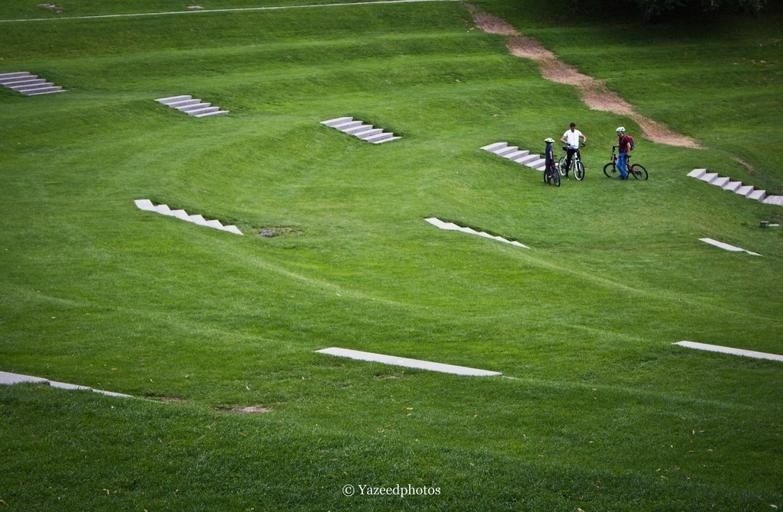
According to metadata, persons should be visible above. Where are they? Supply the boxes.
[614,125,630,180]
[559,121,585,178]
[543,137,555,186]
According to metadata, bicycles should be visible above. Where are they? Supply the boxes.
[540,143,586,187]
[603,146,648,181]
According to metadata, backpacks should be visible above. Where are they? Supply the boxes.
[622,135,635,151]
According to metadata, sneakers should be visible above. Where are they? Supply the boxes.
[549,175,630,186]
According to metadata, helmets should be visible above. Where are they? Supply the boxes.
[615,127,625,132]
[544,138,555,143]
